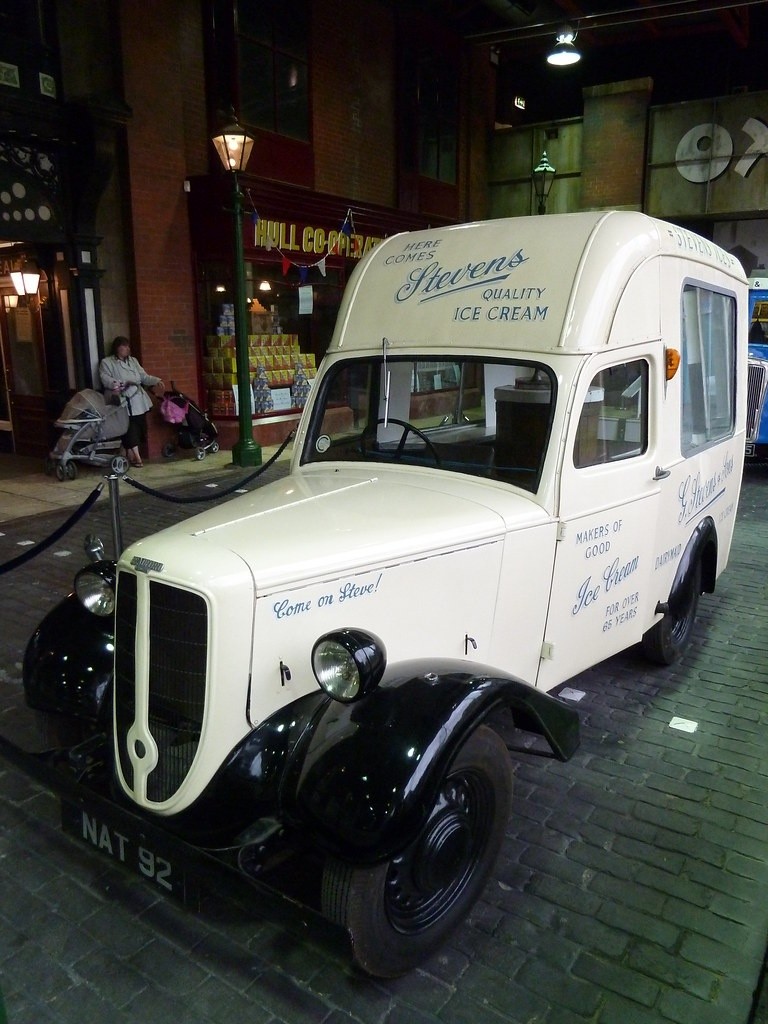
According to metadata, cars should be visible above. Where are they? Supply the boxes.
[22,218,768,981]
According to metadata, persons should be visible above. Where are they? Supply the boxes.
[99,336,165,468]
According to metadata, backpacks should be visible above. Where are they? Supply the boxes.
[160,396,188,424]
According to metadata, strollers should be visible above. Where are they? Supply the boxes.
[40,379,141,482]
[148,381,219,461]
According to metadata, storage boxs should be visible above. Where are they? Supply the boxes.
[202,335,317,417]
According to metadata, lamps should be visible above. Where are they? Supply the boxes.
[9,254,41,296]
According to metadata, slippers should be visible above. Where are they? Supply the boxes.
[134,462,143,467]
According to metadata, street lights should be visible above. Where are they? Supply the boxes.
[213,105,261,469]
